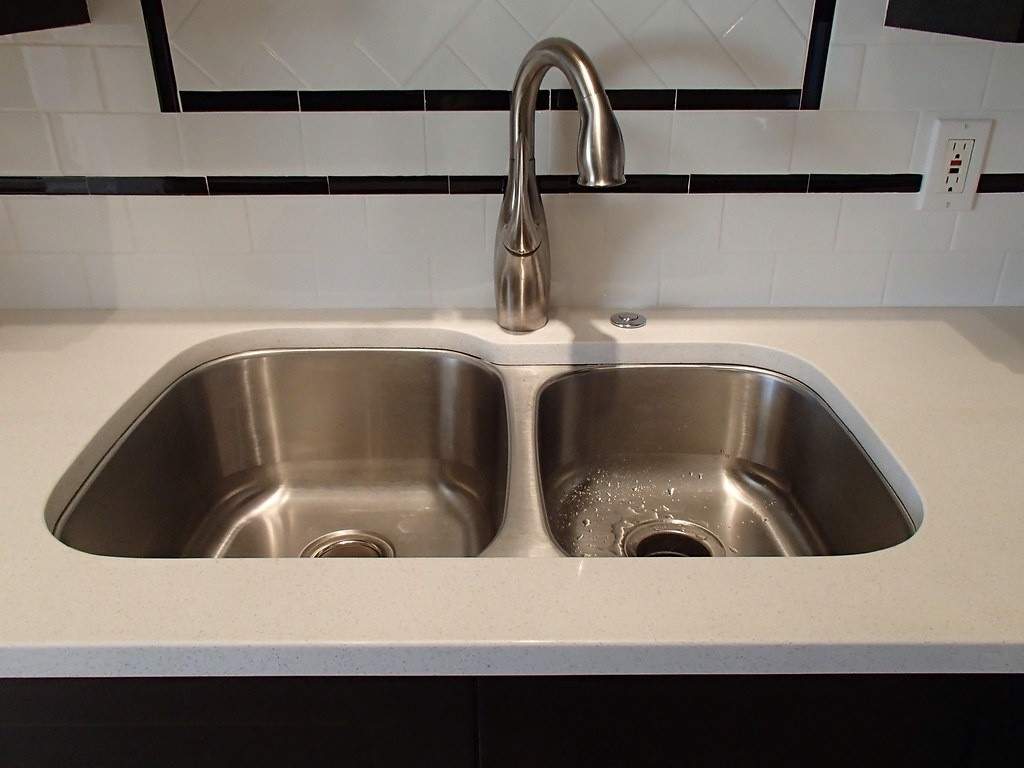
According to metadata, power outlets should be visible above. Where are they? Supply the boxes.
[916,119,993,212]
